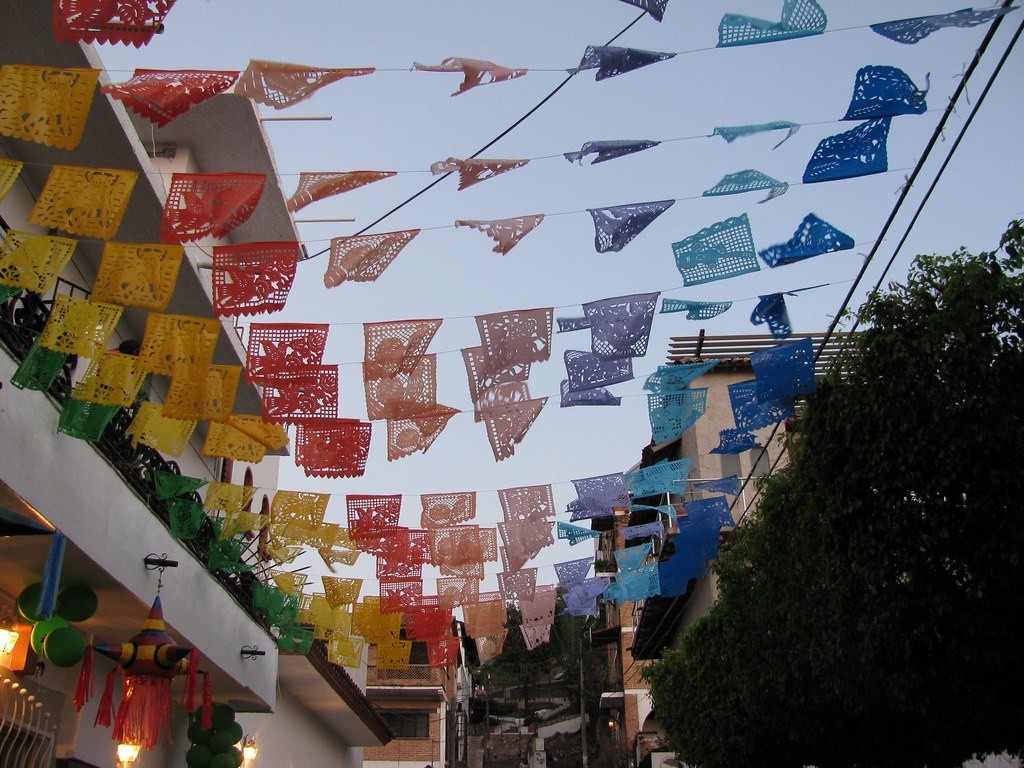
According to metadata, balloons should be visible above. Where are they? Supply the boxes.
[57,584,98,623]
[185,703,244,768]
[16,584,60,623]
[43,627,86,669]
[28,615,69,656]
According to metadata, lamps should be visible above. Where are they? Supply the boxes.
[242,733,259,760]
[0,615,20,655]
[609,720,614,727]
[118,743,143,761]
